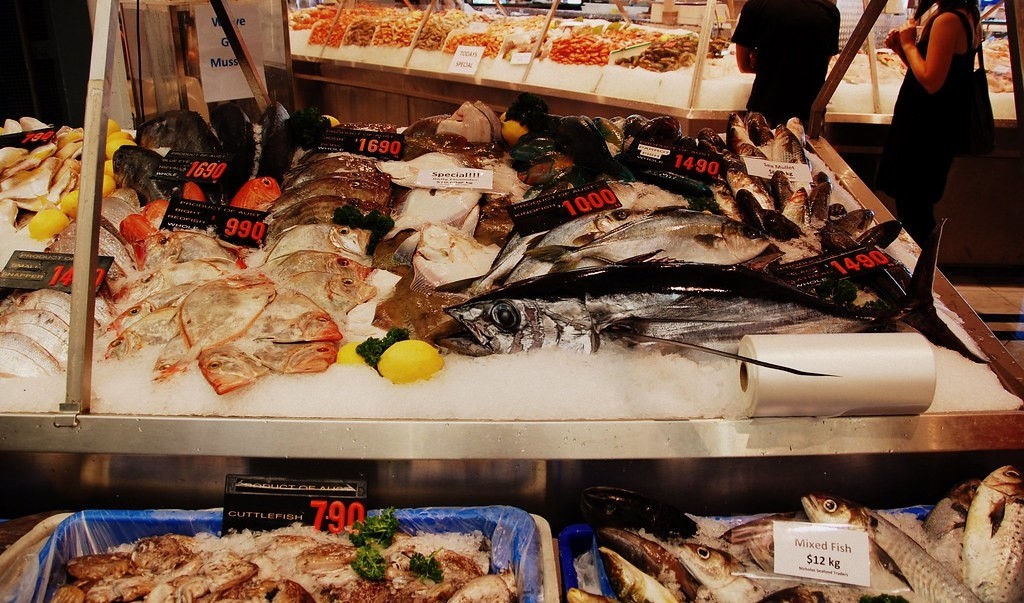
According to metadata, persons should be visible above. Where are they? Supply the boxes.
[876,0,982,249]
[731,0,841,130]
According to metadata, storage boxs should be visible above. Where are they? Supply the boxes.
[558,503,936,603]
[14,505,542,603]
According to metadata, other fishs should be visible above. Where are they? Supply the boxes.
[43,527,520,603]
[565,464,1024,603]
[0,85,994,398]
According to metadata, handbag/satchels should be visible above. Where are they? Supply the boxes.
[927,12,997,158]
